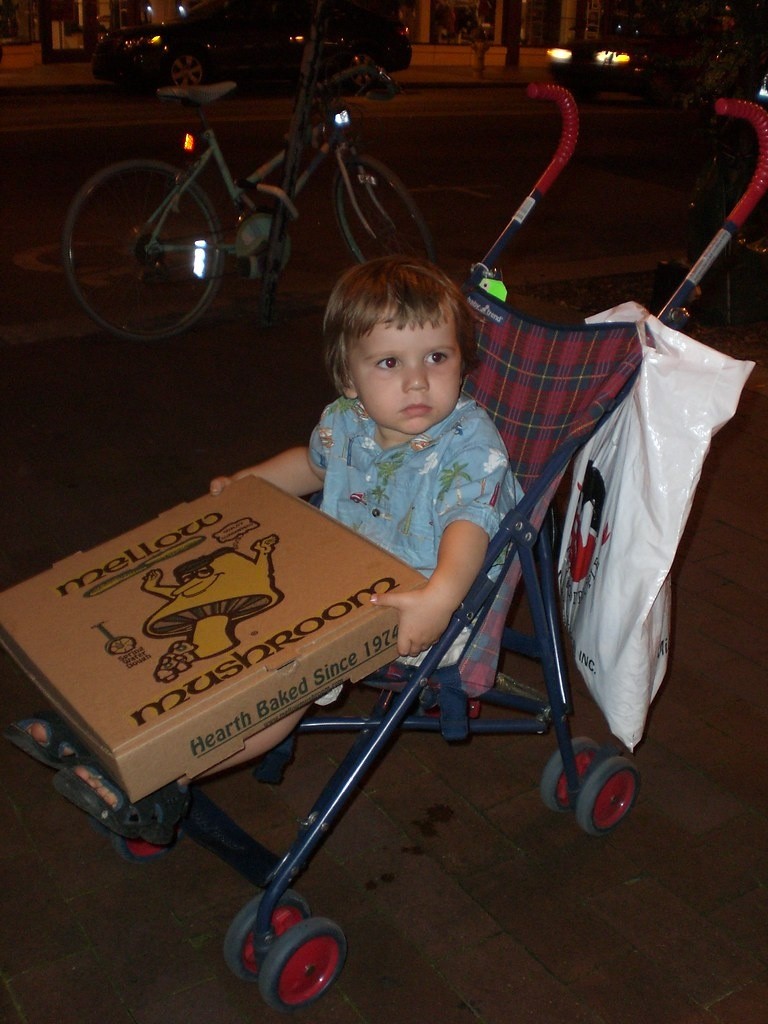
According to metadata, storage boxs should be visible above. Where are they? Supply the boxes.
[0,474,430,803]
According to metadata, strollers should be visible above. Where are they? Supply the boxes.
[2,80,768,1015]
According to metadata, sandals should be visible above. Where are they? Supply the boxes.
[52,759,189,845]
[2,712,88,769]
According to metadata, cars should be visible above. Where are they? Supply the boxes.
[93,0,413,90]
[546,0,759,107]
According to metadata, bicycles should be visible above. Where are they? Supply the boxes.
[53,0,434,342]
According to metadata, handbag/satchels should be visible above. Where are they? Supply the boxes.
[558,299,755,754]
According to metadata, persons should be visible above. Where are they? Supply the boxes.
[0,253,528,846]
